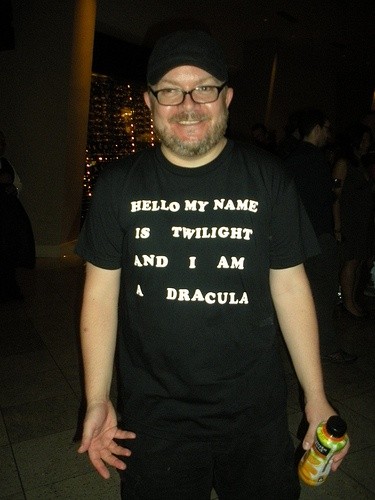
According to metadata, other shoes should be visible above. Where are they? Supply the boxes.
[361,286,375,297]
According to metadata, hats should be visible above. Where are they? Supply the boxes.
[147,27,228,82]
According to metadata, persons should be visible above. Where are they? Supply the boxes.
[76,30,350,500]
[239,95,375,366]
[0,130,36,306]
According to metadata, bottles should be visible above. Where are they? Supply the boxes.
[297,415,349,485]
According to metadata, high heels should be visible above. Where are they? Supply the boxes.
[340,304,370,320]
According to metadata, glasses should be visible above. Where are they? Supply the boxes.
[148,80,227,106]
[323,125,332,132]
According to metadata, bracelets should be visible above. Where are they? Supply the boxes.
[334,224,342,232]
